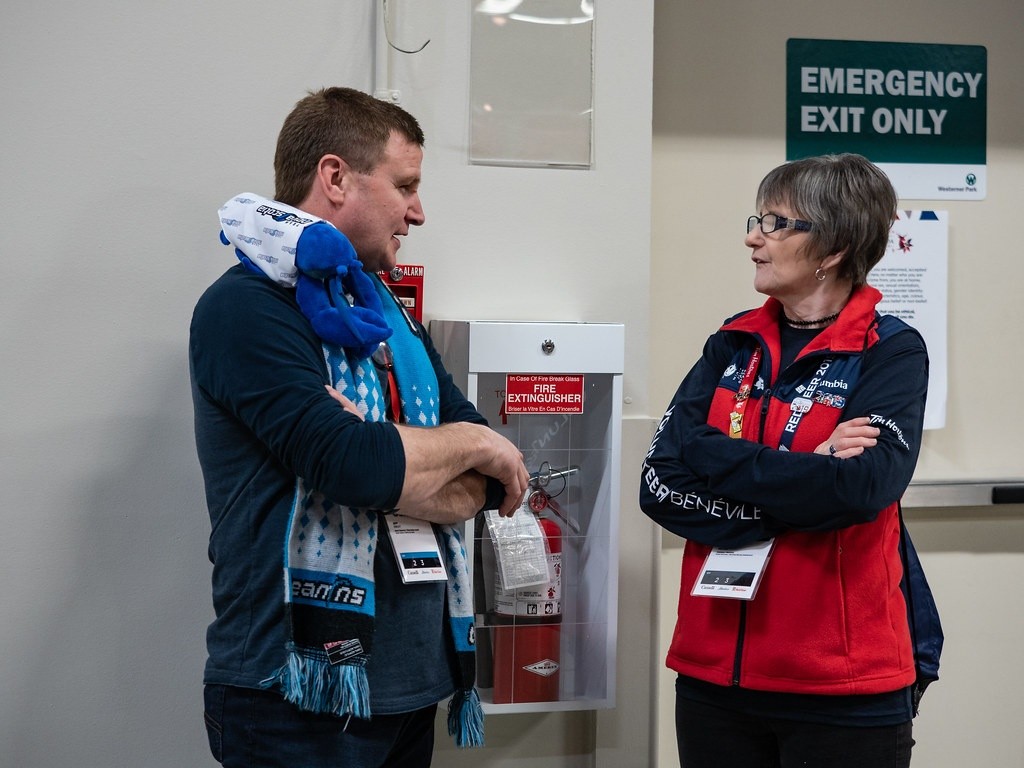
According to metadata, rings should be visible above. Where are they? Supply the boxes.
[831,445,836,454]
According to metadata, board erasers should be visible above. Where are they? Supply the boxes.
[991,485,1024,504]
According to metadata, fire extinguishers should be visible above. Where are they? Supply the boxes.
[470,461,580,703]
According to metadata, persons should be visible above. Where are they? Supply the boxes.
[640,153,944,768]
[188,87,529,768]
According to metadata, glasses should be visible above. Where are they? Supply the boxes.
[746,213,821,235]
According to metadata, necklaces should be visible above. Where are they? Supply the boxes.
[783,309,840,326]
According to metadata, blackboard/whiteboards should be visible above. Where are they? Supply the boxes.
[656,0,1024,509]
